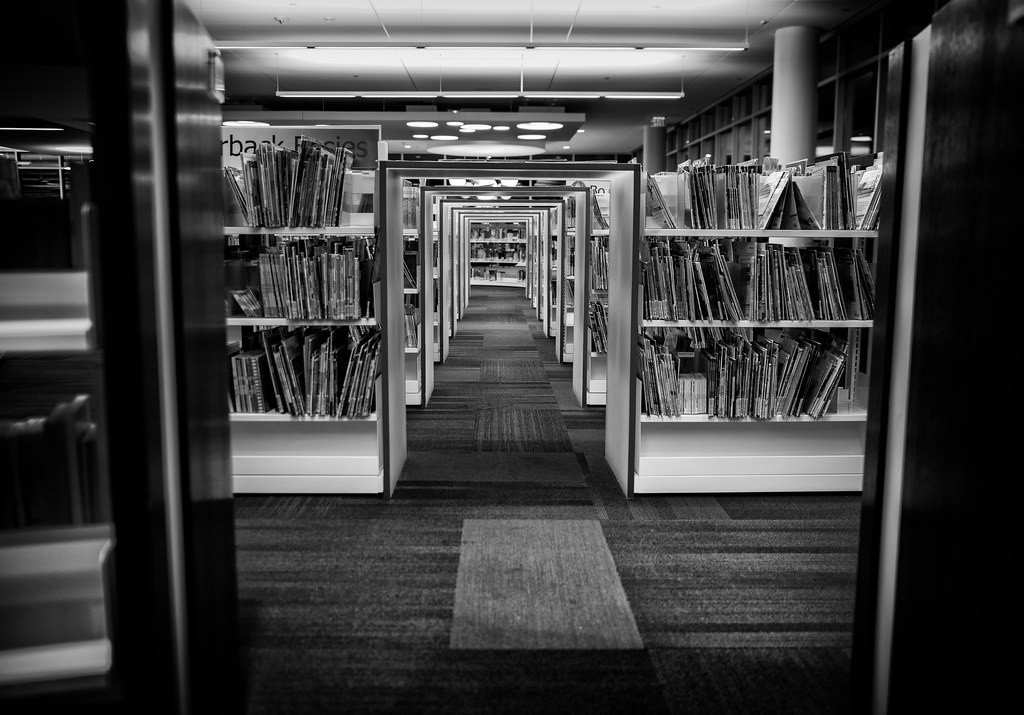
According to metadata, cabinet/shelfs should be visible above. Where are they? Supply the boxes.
[0,272,115,696]
[190,124,469,500]
[470,238,528,287]
[524,165,881,500]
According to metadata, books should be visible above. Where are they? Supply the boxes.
[220,135,437,418]
[469,150,883,421]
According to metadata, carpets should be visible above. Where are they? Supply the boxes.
[448,518,644,651]
